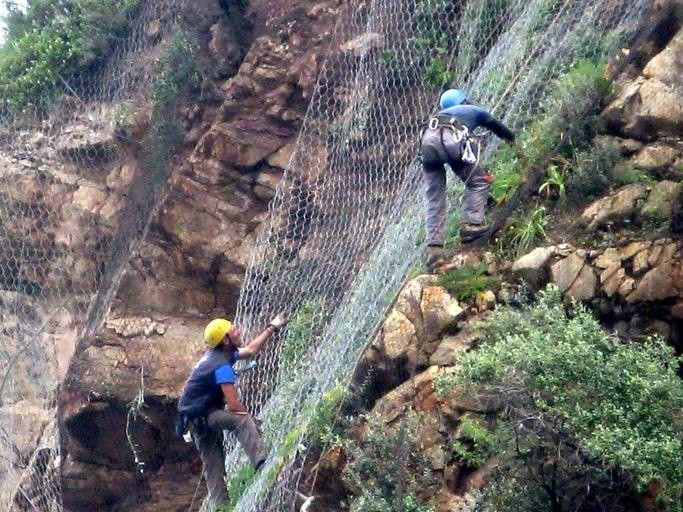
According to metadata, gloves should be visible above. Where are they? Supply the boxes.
[268,310,293,331]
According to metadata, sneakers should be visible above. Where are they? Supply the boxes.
[457,220,490,246]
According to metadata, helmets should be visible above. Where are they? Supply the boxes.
[202,317,234,347]
[437,86,468,108]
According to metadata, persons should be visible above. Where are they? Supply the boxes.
[174,308,292,512]
[418,87,517,269]
